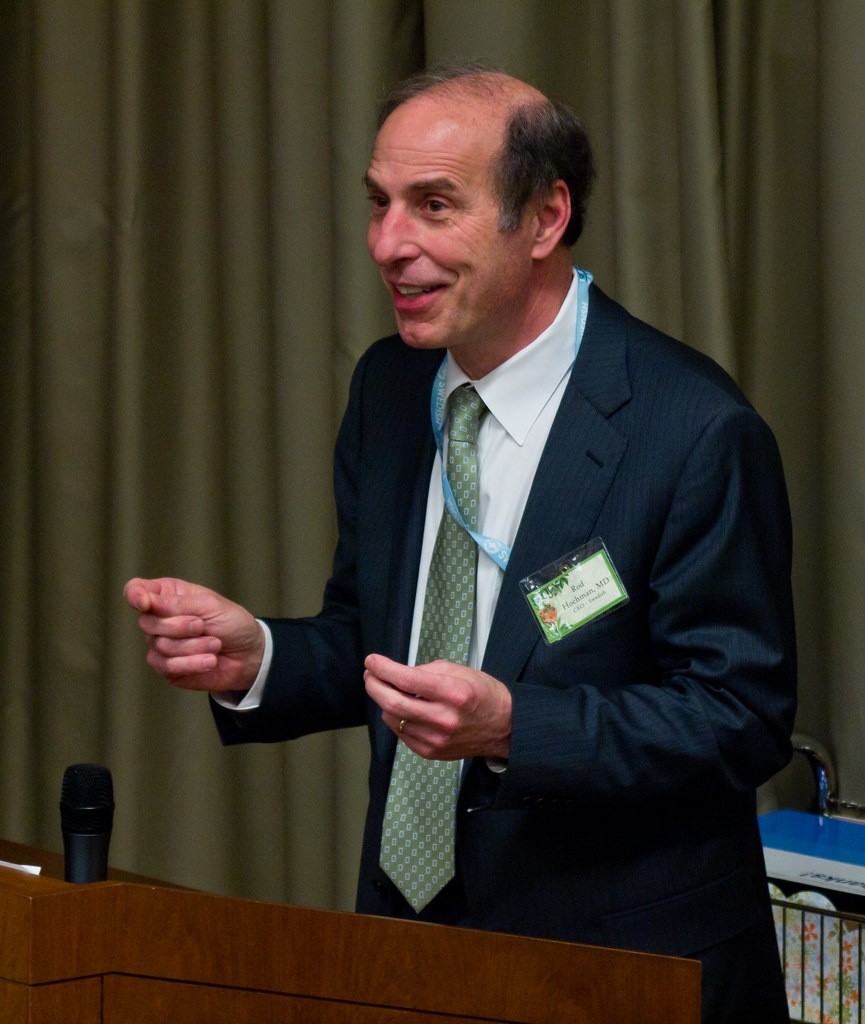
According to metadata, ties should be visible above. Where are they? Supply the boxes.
[379,381,491,906]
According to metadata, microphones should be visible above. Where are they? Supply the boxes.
[59,763,116,884]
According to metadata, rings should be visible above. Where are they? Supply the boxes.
[398,717,407,734]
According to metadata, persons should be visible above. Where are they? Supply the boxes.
[121,70,798,1024]
